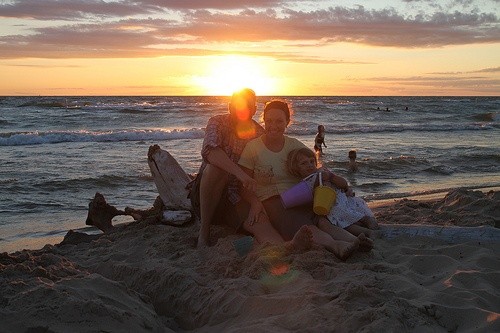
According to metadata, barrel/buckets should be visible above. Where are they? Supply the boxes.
[313,173,336,216]
[280,172,317,209]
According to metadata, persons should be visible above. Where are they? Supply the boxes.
[377,107,380,110]
[404,106,409,111]
[313,125,328,154]
[286,148,383,240]
[185,87,315,259]
[386,107,390,111]
[347,150,358,165]
[237,100,373,260]
